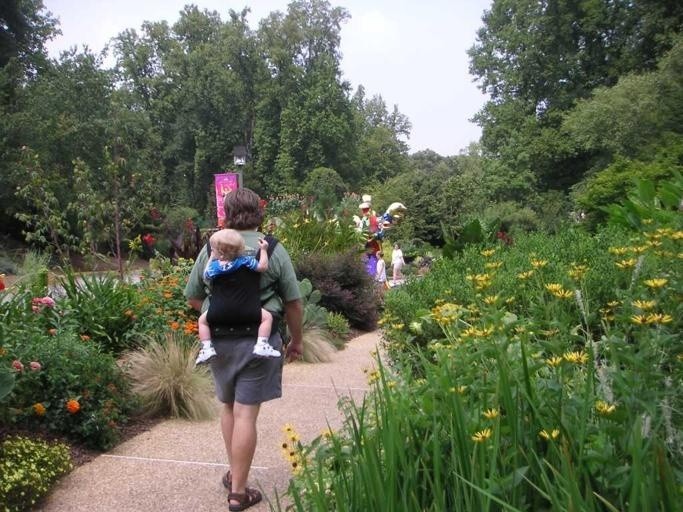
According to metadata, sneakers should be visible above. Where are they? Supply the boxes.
[252,342,281,358]
[195,347,218,366]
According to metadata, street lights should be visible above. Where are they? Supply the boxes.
[231,147,246,188]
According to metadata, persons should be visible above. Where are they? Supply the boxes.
[185,188,302,510]
[390,243,406,288]
[194,226,282,365]
[375,250,388,288]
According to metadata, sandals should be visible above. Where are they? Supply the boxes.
[223,470,232,491]
[228,487,262,511]
